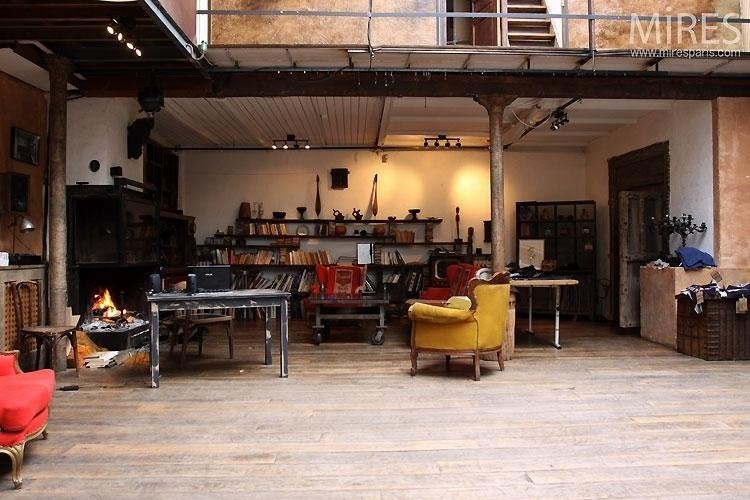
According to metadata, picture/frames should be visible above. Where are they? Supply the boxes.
[11,125,40,166]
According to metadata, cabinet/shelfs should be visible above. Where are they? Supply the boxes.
[675,294,749,360]
[640,264,723,350]
[516,200,596,323]
[196,218,468,270]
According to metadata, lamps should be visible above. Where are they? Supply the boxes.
[424,137,461,149]
[271,138,311,150]
[550,112,570,130]
[7,214,36,257]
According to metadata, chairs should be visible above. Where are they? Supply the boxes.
[0,350,56,489]
[407,270,511,381]
[170,266,235,360]
[9,279,81,381]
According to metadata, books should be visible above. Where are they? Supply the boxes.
[175,223,429,319]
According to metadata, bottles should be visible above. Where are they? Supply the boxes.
[320,282,327,300]
[384,284,387,300]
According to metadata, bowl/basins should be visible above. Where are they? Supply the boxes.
[272,211,286,219]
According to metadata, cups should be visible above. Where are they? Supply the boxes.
[354,229,359,236]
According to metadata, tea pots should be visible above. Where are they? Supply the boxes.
[310,280,320,295]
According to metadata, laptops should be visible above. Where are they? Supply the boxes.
[187,265,232,292]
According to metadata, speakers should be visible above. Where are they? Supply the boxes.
[150,274,161,294]
[188,274,198,294]
[331,168,348,188]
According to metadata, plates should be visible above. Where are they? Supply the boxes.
[296,225,308,236]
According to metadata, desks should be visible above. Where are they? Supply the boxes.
[145,289,292,389]
[509,269,580,349]
